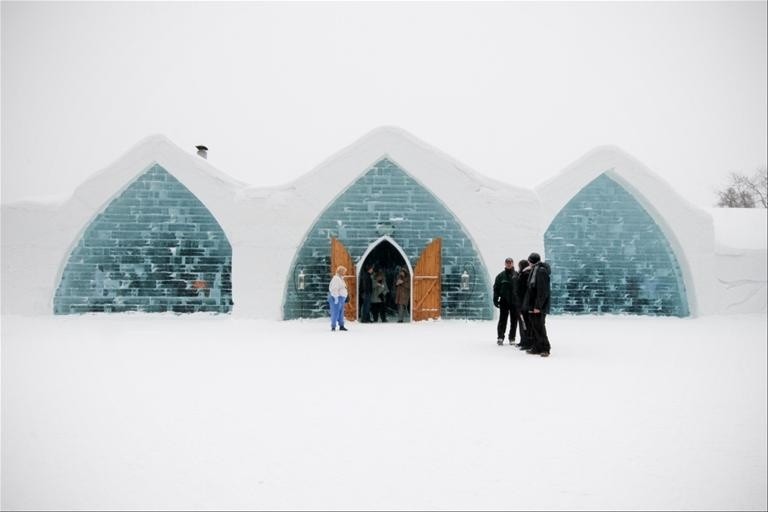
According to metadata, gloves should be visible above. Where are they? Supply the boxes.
[493,299,500,308]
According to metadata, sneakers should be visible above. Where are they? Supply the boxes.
[360,318,404,322]
[497,337,549,357]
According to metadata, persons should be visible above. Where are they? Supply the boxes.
[520,252,551,358]
[512,259,533,349]
[359,262,375,323]
[326,265,351,331]
[492,257,519,346]
[392,269,411,324]
[365,265,390,323]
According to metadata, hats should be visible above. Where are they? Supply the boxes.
[401,264,409,273]
[528,253,540,264]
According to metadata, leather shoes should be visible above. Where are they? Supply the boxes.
[332,327,335,331]
[340,326,347,330]
